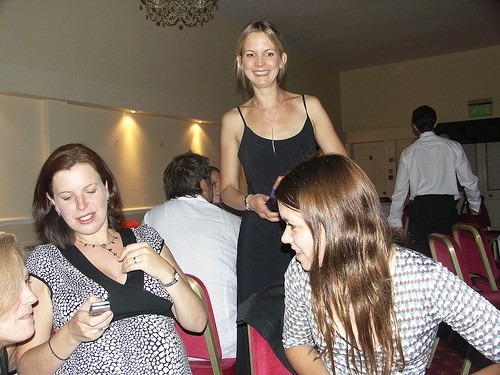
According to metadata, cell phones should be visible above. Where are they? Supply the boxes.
[89,301,111,317]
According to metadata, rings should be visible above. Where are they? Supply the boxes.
[132,257,137,263]
[98,328,104,333]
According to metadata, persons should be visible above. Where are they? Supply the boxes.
[143,153,243,360]
[15,143,208,375]
[220,19,350,375]
[389,106,481,340]
[207,166,243,216]
[1,231,38,375]
[276,154,499,375]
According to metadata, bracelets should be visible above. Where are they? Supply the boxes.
[48,337,70,362]
[243,193,252,211]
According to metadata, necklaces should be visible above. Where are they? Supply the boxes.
[74,229,119,259]
[252,88,283,153]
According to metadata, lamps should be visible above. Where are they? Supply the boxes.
[138,0,219,30]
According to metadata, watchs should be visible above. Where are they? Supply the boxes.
[163,267,180,287]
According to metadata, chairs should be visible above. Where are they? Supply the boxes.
[175,222,500,375]
